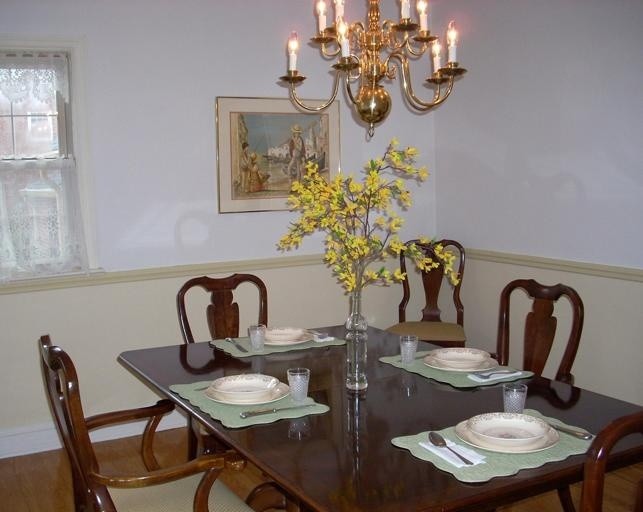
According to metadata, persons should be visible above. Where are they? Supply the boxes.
[245,151,269,193]
[286,122,306,195]
[238,141,250,191]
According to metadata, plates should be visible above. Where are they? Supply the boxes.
[204,380,289,406]
[261,335,313,345]
[423,354,499,372]
[455,419,560,454]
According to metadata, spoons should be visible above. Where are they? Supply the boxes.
[473,370,516,379]
[307,331,328,339]
[428,432,473,465]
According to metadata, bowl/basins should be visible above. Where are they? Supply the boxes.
[431,347,491,368]
[211,374,279,399]
[466,413,552,446]
[265,326,305,341]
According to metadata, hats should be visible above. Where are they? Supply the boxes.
[291,124,303,133]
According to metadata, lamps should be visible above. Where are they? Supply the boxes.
[277,0,466,137]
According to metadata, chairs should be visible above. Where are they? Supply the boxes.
[580,415,643,512]
[37,333,250,512]
[173,269,268,461]
[381,237,467,348]
[491,274,585,512]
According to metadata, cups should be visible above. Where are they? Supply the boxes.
[399,334,419,365]
[248,324,265,352]
[287,416,311,439]
[394,370,417,400]
[286,368,310,403]
[501,382,528,414]
[250,355,266,374]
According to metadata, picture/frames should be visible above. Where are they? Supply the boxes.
[214,96,341,214]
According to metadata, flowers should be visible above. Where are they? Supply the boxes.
[277,136,458,297]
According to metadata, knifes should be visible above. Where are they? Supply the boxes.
[237,403,315,418]
[224,337,248,352]
[397,353,429,363]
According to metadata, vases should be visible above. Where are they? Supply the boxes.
[342,263,368,391]
